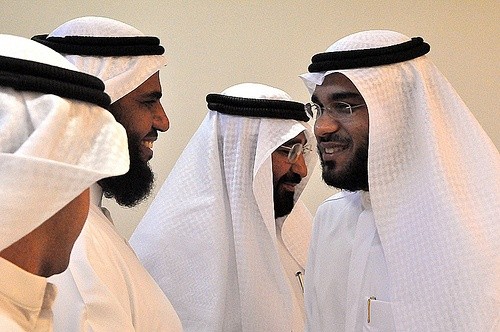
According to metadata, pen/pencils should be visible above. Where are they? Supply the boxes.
[296,272,306,293]
[367,296,378,323]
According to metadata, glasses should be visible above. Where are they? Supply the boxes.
[276,142,317,165]
[304,97,367,123]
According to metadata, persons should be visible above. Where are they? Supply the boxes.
[0,33,130,332]
[303,30,500,332]
[125,81,313,332]
[29,16,182,332]
